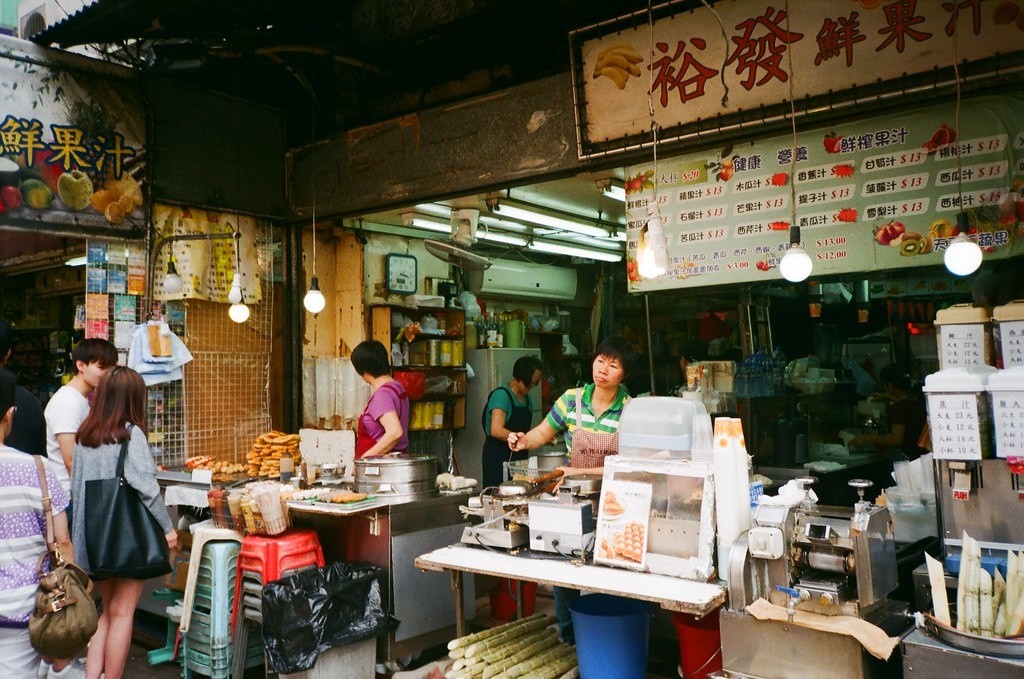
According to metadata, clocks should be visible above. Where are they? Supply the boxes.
[386,253,417,293]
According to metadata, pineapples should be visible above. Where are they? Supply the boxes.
[65,103,105,190]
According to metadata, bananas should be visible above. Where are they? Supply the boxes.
[593,42,644,90]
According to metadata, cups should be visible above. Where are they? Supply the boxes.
[466,321,477,350]
[506,320,525,348]
[280,457,294,485]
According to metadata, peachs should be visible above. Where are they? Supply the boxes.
[720,159,734,182]
[876,222,906,245]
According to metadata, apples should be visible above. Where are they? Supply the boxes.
[57,171,95,212]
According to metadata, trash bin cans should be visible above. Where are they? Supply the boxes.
[262,559,383,679]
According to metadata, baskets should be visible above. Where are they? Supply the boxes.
[507,460,560,481]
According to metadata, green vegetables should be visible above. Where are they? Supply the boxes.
[963,202,1019,246]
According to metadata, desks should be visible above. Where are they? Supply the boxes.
[412,542,726,638]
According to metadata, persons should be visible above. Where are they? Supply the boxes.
[667,339,709,397]
[43,337,119,541]
[0,320,48,458]
[848,362,931,462]
[69,365,178,679]
[507,335,633,515]
[481,356,558,489]
[0,366,75,679]
[350,340,410,460]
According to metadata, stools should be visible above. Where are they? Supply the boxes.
[146,526,325,679]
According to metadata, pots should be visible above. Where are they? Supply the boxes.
[350,453,439,501]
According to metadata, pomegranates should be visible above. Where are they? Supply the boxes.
[921,123,955,153]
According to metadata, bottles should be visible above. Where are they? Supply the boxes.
[876,489,886,506]
[298,457,318,491]
[435,312,445,329]
[471,312,513,348]
[241,482,285,534]
[409,338,464,367]
[409,401,444,429]
[206,485,245,531]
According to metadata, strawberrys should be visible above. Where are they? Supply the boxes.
[824,132,843,152]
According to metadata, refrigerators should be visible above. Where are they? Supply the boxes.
[452,349,542,491]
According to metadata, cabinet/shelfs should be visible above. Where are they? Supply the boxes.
[368,304,466,432]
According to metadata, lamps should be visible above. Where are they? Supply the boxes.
[401,178,625,260]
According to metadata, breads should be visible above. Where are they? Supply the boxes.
[599,521,645,563]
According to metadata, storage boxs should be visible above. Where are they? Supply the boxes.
[718,609,864,679]
[922,301,1024,460]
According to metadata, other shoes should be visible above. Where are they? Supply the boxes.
[37,659,52,679]
[47,659,105,679]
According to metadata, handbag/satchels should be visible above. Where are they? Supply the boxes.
[28,455,97,659]
[85,423,174,580]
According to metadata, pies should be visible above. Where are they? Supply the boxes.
[246,430,300,478]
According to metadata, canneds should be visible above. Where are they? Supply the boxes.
[425,339,464,367]
[411,401,444,429]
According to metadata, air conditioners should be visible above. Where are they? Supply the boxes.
[466,256,578,302]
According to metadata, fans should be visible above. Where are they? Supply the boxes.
[425,208,492,272]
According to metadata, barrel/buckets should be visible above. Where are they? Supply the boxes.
[672,608,723,679]
[567,593,650,679]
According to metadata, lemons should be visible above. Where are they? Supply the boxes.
[93,191,136,222]
[19,178,52,210]
[926,219,953,253]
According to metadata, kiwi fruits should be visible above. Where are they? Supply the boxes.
[899,231,931,256]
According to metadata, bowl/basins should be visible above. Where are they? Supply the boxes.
[322,463,347,480]
[946,554,1007,578]
[421,313,438,330]
